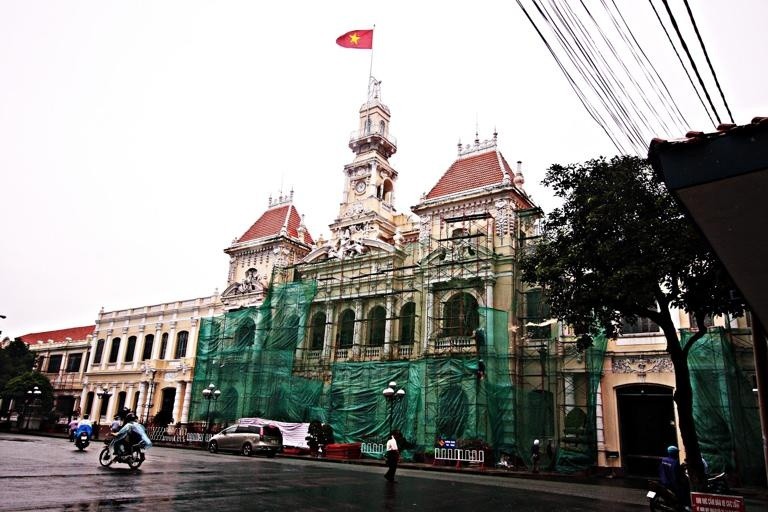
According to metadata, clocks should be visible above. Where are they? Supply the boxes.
[356,181,366,193]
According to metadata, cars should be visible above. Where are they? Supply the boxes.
[208,424,283,458]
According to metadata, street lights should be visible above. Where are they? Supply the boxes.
[25,386,42,430]
[201,384,221,448]
[97,385,112,425]
[383,382,405,436]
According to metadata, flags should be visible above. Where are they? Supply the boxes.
[335,29,373,49]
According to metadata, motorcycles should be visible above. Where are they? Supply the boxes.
[70,430,89,451]
[100,432,146,469]
[647,463,728,512]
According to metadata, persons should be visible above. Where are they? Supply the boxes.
[659,444,692,511]
[92,421,103,440]
[74,414,92,445]
[384,430,401,481]
[109,415,121,431]
[68,416,77,437]
[104,413,153,461]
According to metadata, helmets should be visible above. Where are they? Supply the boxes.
[125,412,138,419]
[666,445,678,456]
